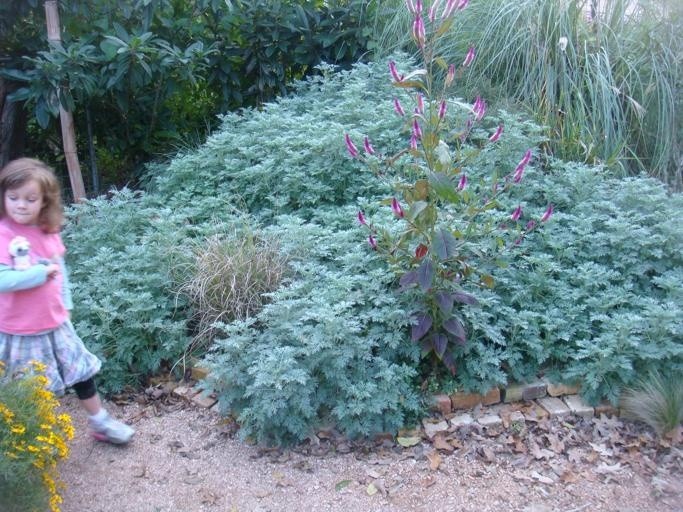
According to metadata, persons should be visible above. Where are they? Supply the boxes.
[0,155,136,446]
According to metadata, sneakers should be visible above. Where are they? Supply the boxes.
[86,410,135,445]
